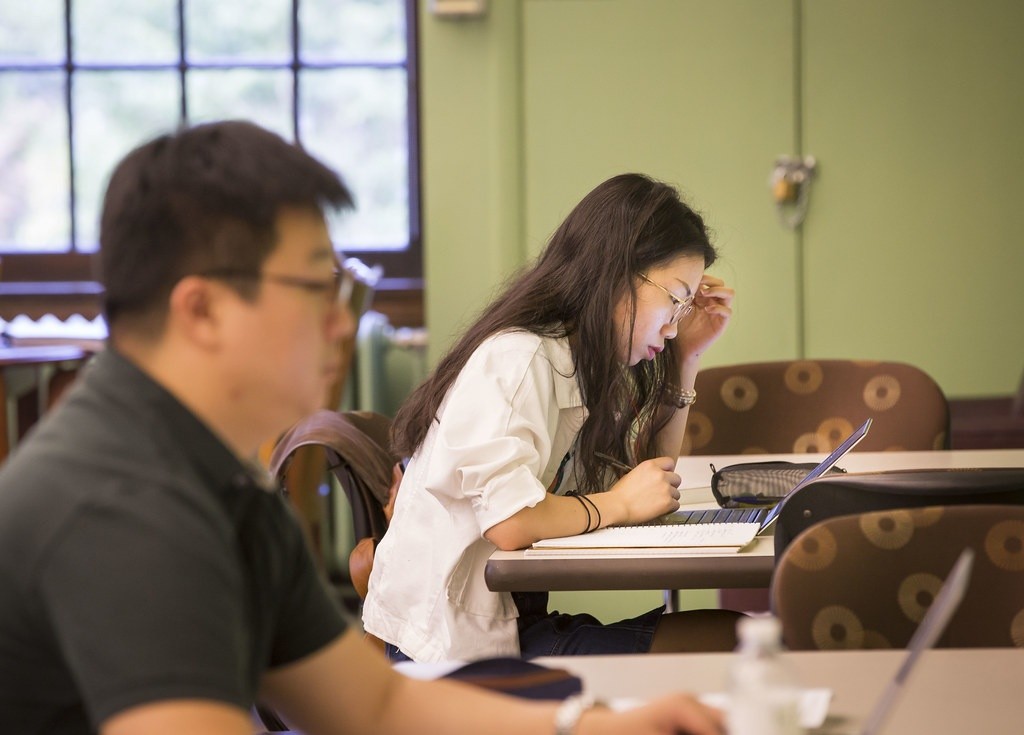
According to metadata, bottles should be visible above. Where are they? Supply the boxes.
[722,618,802,735]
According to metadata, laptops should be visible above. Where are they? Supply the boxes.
[531,417,871,549]
[852,546,975,734]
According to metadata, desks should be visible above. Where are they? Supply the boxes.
[484,448,1024,593]
[385,648,1024,735]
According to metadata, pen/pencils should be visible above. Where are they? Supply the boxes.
[593,450,632,471]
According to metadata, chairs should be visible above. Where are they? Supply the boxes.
[678,360,1023,651]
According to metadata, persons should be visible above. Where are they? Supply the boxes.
[4,122,727,733]
[359,168,766,652]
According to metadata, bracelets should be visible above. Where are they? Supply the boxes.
[563,488,601,533]
[552,688,609,729]
[657,378,698,407]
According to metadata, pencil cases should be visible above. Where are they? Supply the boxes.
[710,461,848,509]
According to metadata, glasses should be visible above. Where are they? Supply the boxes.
[157,256,354,321]
[637,272,694,325]
